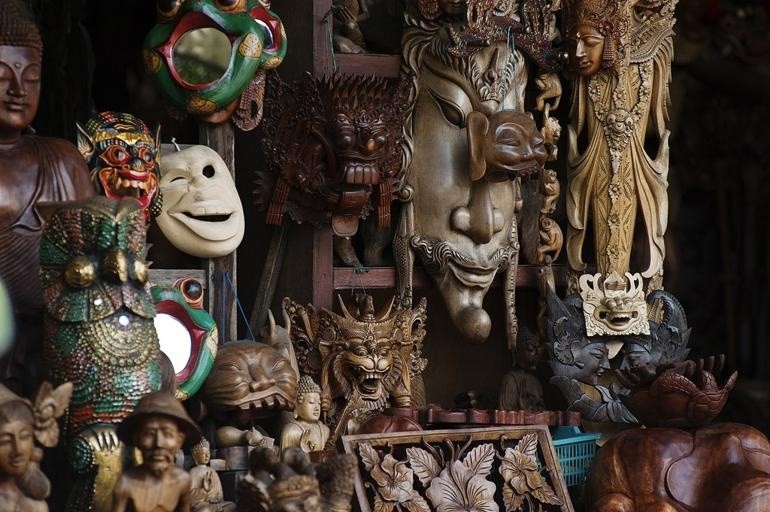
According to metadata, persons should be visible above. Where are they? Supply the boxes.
[499,314,678,426]
[552,1,639,80]
[1,0,99,397]
[1,375,358,512]
